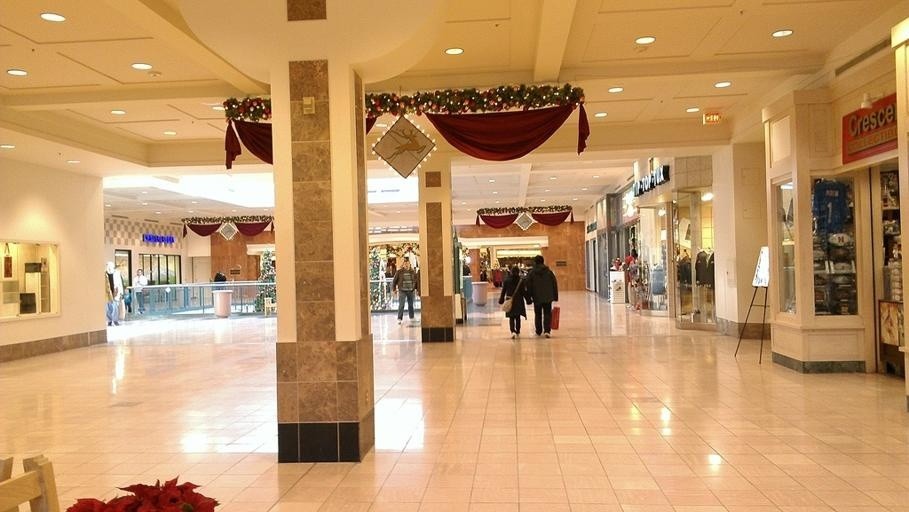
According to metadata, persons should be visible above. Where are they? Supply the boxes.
[527,255,559,339]
[463,259,471,276]
[214,272,228,289]
[123,289,131,313]
[498,266,532,339]
[392,260,416,325]
[103,261,124,325]
[131,269,149,314]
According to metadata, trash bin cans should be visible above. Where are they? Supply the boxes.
[211,290,234,318]
[471,282,489,307]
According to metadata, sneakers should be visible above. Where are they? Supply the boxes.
[108,322,112,326]
[516,333,519,337]
[410,318,420,323]
[544,332,552,338]
[114,322,120,326]
[397,318,402,324]
[510,332,516,339]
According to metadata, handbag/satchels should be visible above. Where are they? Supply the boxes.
[550,301,561,330]
[499,295,513,312]
[118,296,126,320]
